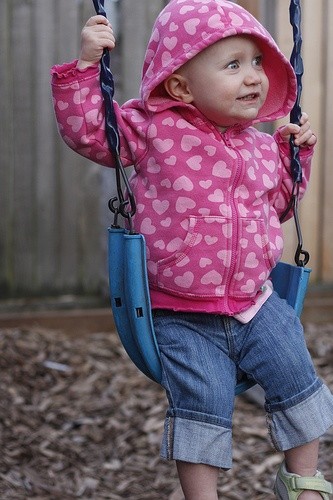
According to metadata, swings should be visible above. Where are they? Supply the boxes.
[88,0,313,397]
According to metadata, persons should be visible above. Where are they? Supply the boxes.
[48,0,333,500]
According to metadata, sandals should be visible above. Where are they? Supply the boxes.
[272,457,333,500]
